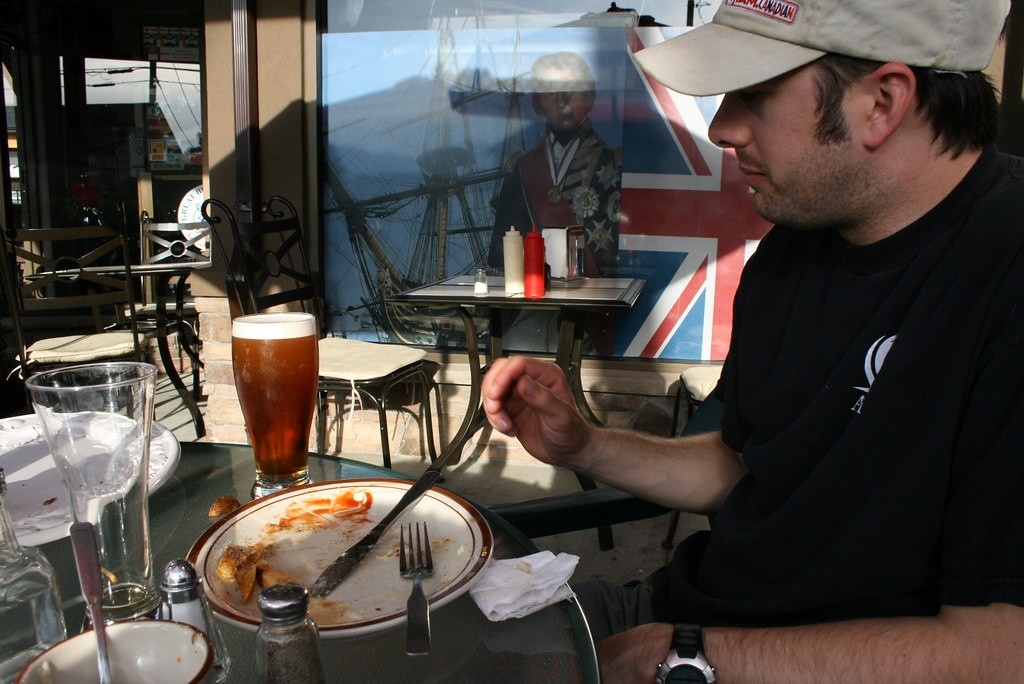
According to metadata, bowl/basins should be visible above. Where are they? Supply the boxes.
[16,620,214,684]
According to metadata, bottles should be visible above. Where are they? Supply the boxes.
[254,583,326,684]
[158,558,231,684]
[524,225,545,298]
[0,470,69,684]
[503,226,525,294]
[474,268,489,296]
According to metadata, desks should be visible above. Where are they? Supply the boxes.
[383,273,648,492]
[24,262,213,438]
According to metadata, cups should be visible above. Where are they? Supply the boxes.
[25,362,161,622]
[231,312,320,500]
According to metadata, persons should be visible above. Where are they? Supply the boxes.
[480,0,1024,684]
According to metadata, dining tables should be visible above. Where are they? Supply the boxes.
[0,441,601,684]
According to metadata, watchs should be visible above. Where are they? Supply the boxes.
[654,623,717,684]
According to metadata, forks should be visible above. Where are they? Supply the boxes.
[400,521,431,655]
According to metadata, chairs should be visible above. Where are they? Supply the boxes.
[201,196,449,482]
[660,365,725,549]
[0,200,159,422]
[121,209,220,402]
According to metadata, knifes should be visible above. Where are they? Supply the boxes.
[309,471,442,599]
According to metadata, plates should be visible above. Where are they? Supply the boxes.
[0,412,181,548]
[186,478,494,639]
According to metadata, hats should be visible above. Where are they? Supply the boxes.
[632,0,1012,97]
